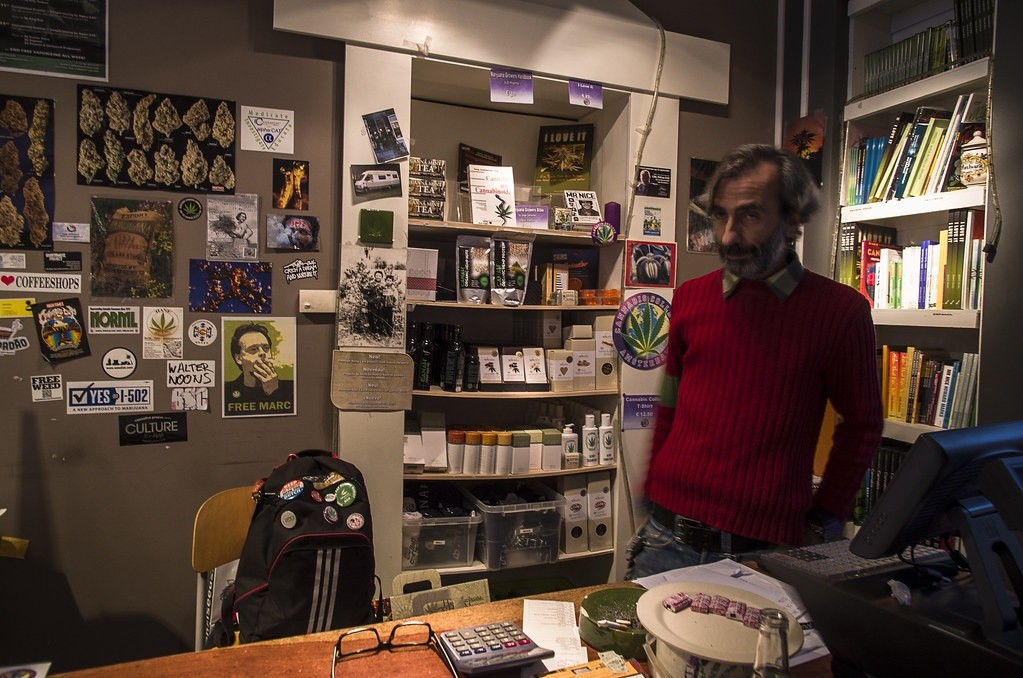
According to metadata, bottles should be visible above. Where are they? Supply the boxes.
[751,608,790,678]
[525,397,615,467]
[446,423,512,475]
[406,319,479,393]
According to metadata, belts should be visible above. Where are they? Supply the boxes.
[651,502,778,556]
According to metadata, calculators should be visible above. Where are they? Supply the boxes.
[437,620,558,675]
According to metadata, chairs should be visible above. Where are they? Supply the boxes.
[191,485,260,653]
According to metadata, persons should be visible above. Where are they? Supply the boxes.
[625,143,883,581]
[635,170,658,195]
[367,271,398,337]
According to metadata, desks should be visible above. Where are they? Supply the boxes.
[45,558,834,675]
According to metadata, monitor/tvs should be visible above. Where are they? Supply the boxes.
[849,419,1023,560]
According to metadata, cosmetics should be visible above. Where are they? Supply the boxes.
[409,320,480,392]
[444,399,617,476]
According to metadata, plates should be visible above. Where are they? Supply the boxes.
[638,582,805,664]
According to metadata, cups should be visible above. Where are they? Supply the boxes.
[960,131,987,187]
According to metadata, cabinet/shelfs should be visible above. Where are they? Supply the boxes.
[401,216,623,596]
[830,3,1023,533]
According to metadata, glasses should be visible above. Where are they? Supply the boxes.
[330,624,460,678]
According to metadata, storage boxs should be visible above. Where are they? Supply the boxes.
[450,479,567,569]
[400,491,483,570]
[511,312,620,475]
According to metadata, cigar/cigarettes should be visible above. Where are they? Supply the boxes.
[597,620,627,631]
[616,620,631,625]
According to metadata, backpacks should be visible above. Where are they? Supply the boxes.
[203,448,377,651]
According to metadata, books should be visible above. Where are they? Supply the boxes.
[533,124,594,197]
[851,343,979,526]
[564,190,603,225]
[839,209,984,309]
[457,143,502,192]
[467,164,516,227]
[843,0,995,206]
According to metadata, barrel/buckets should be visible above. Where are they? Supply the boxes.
[641,632,754,678]
